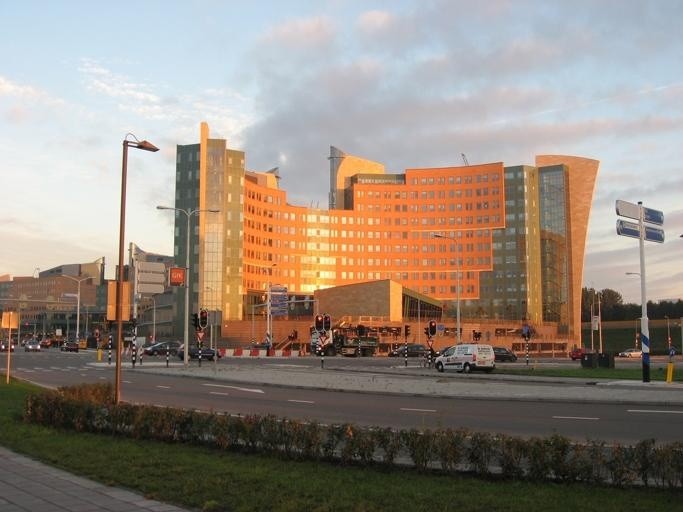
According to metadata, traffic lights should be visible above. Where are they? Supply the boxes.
[422,327,428,336]
[315,314,323,331]
[198,309,208,329]
[428,320,437,336]
[404,324,411,337]
[324,314,331,331]
[191,313,197,330]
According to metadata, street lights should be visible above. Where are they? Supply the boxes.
[589,301,601,351]
[624,272,641,277]
[266,261,278,335]
[61,272,97,340]
[154,203,222,366]
[141,296,156,343]
[663,315,670,351]
[634,317,641,348]
[433,231,461,343]
[114,129,160,400]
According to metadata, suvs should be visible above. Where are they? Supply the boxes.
[176,342,223,362]
[492,345,517,362]
[386,343,425,358]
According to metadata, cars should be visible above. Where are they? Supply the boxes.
[617,348,642,358]
[567,347,592,361]
[0,329,65,353]
[143,339,182,357]
[60,342,79,353]
[433,345,452,358]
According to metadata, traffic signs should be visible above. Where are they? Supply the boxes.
[643,206,665,226]
[614,218,640,239]
[271,302,289,307]
[270,286,288,293]
[612,198,640,221]
[643,228,666,244]
[270,294,288,300]
[271,309,288,316]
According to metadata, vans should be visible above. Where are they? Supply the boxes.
[434,343,496,375]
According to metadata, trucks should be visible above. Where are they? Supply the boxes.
[309,326,377,357]
